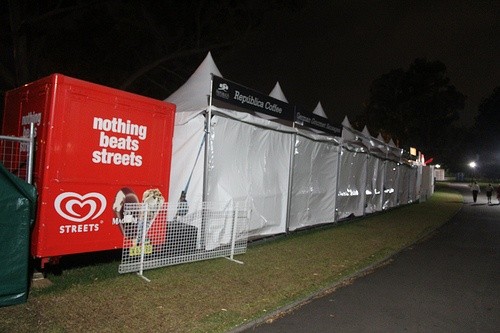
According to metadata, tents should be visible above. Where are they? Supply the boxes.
[160,51,435,254]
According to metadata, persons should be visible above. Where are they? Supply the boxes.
[485,183,494,205]
[470,181,481,204]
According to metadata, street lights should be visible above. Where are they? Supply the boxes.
[468,161,478,185]
